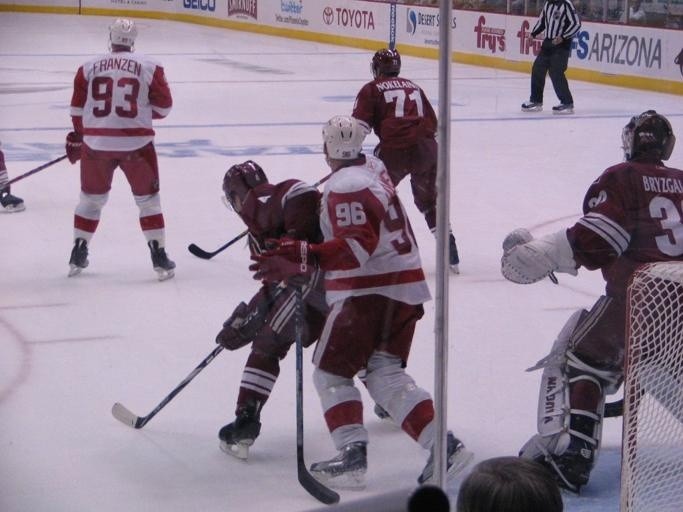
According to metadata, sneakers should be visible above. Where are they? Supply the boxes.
[69,238,89,268]
[553,102,573,110]
[450,233,459,265]
[219,400,261,445]
[148,240,175,269]
[310,441,367,472]
[0,187,24,208]
[417,431,465,484]
[534,448,592,485]
[522,101,543,108]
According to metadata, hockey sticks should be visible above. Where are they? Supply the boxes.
[294,274,339,505]
[112,272,291,428]
[603,384,643,416]
[188,168,338,259]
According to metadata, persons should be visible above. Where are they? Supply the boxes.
[456,456,564,512]
[522,1,581,112]
[262,116,464,484]
[392,0,683,31]
[350,49,459,266]
[500,109,683,496]
[69,18,176,272]
[219,159,392,446]
[0,150,24,208]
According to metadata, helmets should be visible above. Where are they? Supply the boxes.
[620,110,676,161]
[222,160,268,214]
[109,18,138,47]
[370,48,401,80]
[322,115,363,160]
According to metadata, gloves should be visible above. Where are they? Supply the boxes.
[500,227,578,284]
[248,237,314,286]
[65,132,83,164]
[215,302,253,351]
